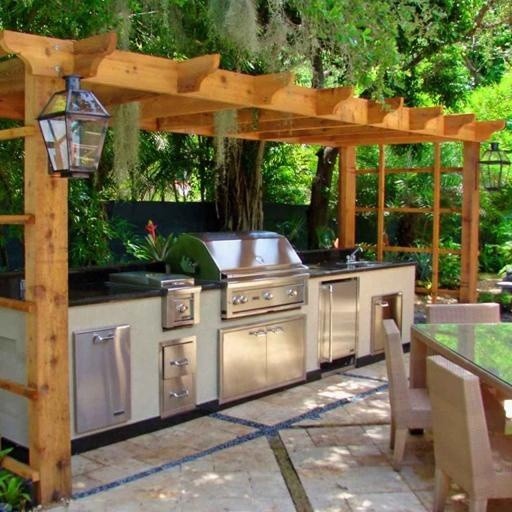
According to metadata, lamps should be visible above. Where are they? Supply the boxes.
[35,75,111,179]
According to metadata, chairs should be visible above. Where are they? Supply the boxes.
[426,355,512,512]
[408,322,512,436]
[382,319,433,471]
[427,303,501,355]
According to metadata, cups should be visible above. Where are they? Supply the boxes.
[69,271,80,289]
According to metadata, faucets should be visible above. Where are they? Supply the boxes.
[350,246,364,260]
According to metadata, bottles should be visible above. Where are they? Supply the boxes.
[16,273,24,299]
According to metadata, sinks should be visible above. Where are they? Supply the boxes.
[346,260,393,269]
